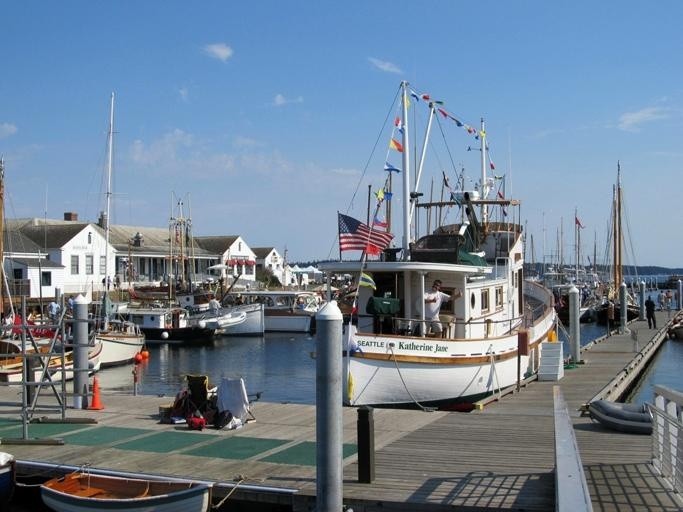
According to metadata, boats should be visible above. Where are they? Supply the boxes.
[0,448,14,506]
[583,396,681,432]
[658,274,683,291]
[667,311,683,341]
[40,470,218,512]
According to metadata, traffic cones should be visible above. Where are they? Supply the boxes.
[86,375,105,411]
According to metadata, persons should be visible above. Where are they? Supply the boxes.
[665,288,673,312]
[424,280,462,338]
[658,291,667,311]
[645,295,659,329]
[47,300,60,319]
[67,295,73,315]
[102,272,121,290]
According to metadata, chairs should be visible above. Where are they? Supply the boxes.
[185,374,263,424]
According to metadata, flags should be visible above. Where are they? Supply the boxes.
[339,213,395,251]
[373,90,508,227]
[359,272,377,290]
